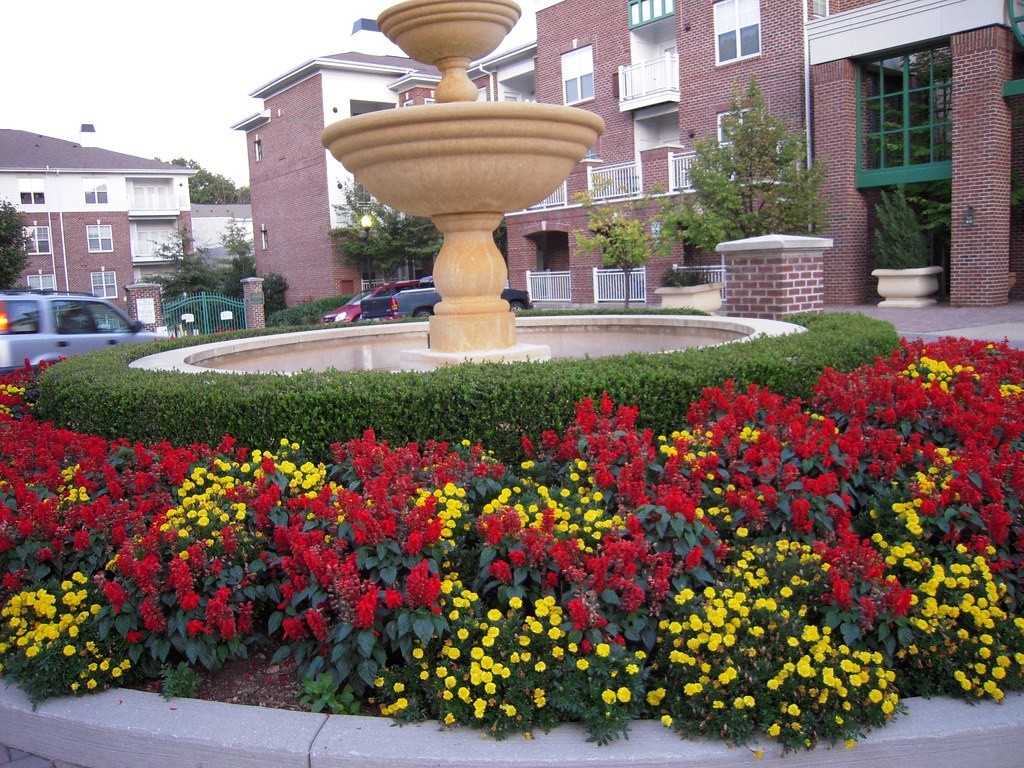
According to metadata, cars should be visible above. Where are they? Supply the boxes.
[319,279,419,324]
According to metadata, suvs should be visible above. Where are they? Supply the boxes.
[1,287,173,376]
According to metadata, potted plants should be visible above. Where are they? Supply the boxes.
[655,260,723,317]
[871,185,944,308]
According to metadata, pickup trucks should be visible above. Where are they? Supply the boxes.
[360,281,534,322]
